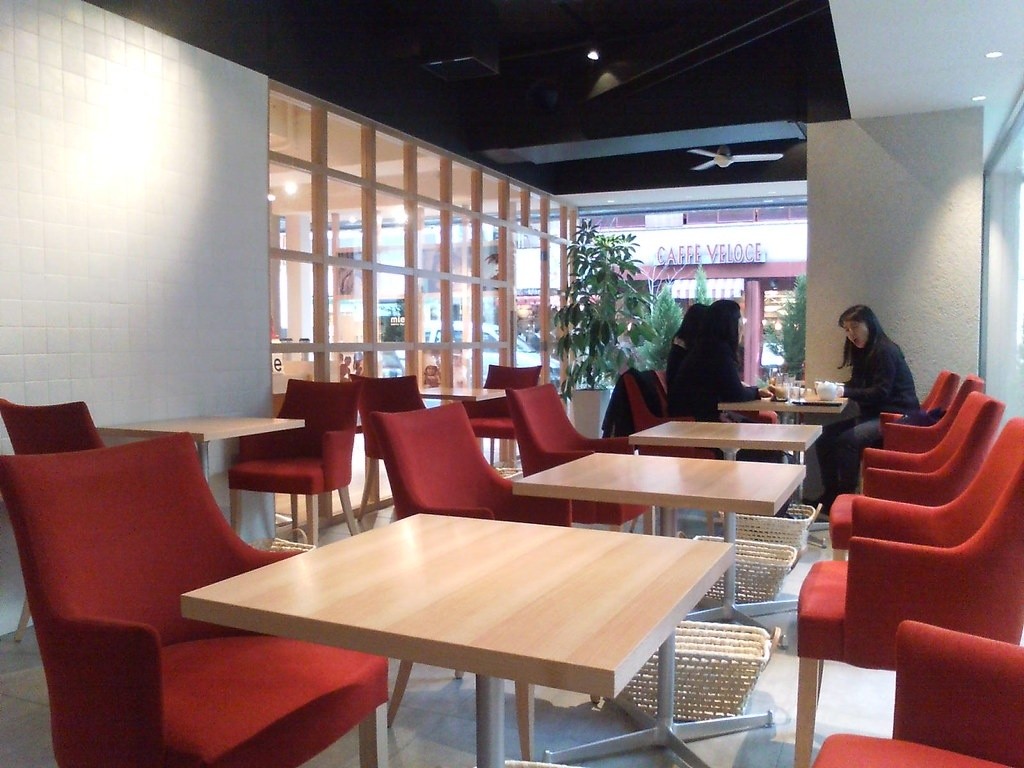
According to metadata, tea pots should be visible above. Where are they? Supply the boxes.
[815,381,839,400]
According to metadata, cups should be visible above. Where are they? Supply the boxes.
[776,373,788,401]
[769,368,781,386]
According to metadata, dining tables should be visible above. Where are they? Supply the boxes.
[419,387,507,402]
[628,420,823,651]
[718,386,848,549]
[180,513,740,768]
[97,416,305,484]
[514,452,806,768]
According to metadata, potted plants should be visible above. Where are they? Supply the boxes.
[554,219,661,441]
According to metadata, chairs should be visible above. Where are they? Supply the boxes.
[880,370,961,427]
[506,383,656,536]
[368,401,572,760]
[812,619,1024,768]
[349,373,429,522]
[0,397,107,642]
[0,432,388,768]
[228,378,358,548]
[794,417,1024,767]
[829,390,1006,549]
[462,364,542,468]
[648,370,667,414]
[623,370,720,537]
[880,375,985,452]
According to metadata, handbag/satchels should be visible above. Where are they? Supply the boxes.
[895,407,946,427]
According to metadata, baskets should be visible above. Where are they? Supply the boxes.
[719,503,824,555]
[693,536,797,608]
[618,621,781,721]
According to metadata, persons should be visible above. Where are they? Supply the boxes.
[339,353,363,381]
[422,357,443,390]
[667,304,707,397]
[810,303,921,516]
[674,299,784,463]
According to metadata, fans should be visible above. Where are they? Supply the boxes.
[687,145,784,170]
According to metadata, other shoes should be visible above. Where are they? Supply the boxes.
[802,494,833,522]
